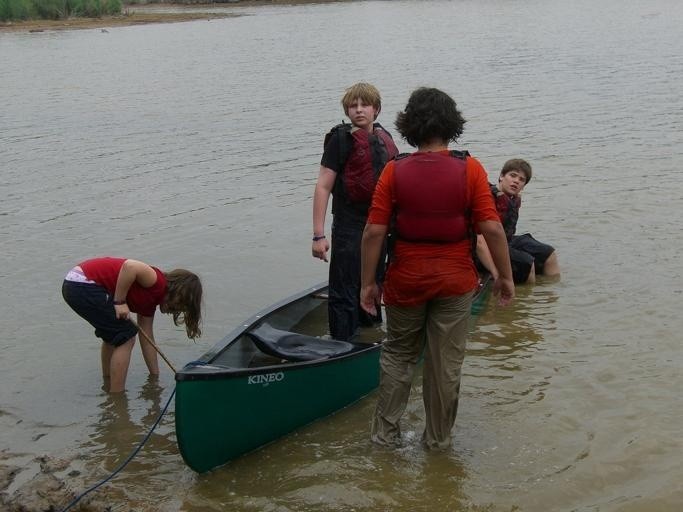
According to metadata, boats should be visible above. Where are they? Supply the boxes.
[174,269,493,475]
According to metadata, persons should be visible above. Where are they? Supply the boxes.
[311,82,399,344]
[62,256,203,393]
[359,86,515,455]
[474,158,561,285]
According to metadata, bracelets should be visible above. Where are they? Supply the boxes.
[312,236,325,241]
[112,301,126,306]
